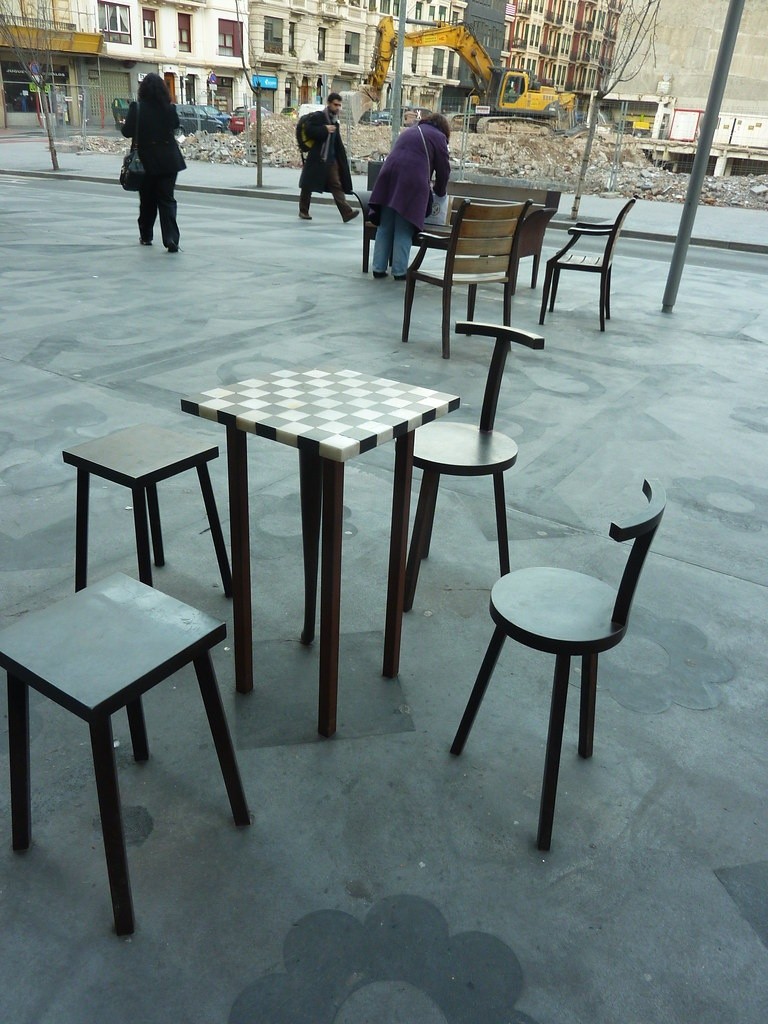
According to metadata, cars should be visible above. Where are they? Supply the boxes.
[383,107,434,117]
[280,107,299,119]
[229,107,274,135]
[197,105,232,131]
[358,110,395,127]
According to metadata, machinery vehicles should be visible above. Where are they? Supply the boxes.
[336,15,577,138]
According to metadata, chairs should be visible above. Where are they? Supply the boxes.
[539,199,637,331]
[401,198,533,360]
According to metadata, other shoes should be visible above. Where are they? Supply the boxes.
[343,210,359,222]
[168,240,178,253]
[299,211,312,220]
[373,271,388,278]
[139,238,152,245]
[394,274,407,280]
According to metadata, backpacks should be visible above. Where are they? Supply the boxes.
[296,111,329,152]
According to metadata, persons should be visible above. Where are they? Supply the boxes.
[369,113,452,280]
[297,93,360,223]
[117,71,187,253]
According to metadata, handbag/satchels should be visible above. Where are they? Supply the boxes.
[424,195,454,226]
[120,148,144,191]
[425,190,433,219]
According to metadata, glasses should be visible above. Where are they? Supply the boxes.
[332,102,341,107]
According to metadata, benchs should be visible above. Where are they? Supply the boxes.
[349,180,562,295]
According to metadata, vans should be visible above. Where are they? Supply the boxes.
[172,103,225,137]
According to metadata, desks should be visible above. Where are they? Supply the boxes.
[180,359,460,737]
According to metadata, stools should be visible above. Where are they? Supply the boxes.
[395,321,545,612]
[0,571,252,937]
[61,423,233,598]
[448,478,667,852]
